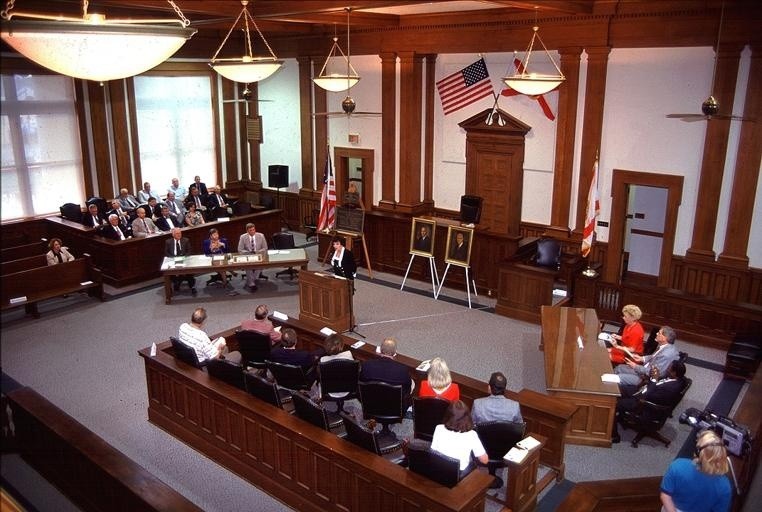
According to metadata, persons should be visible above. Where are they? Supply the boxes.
[416,226,431,252]
[330,236,357,296]
[358,337,412,416]
[418,357,460,401]
[455,232,467,262]
[319,334,354,399]
[270,328,314,375]
[660,430,732,512]
[430,400,489,480]
[605,304,686,443]
[240,304,282,346]
[178,308,227,366]
[46,175,268,296]
[471,372,523,427]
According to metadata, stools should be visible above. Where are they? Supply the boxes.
[733,333,761,348]
[724,343,762,380]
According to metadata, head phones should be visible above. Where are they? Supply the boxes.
[695,441,725,457]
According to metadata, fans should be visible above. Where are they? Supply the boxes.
[310,8,384,118]
[223,28,273,117]
[666,0,759,124]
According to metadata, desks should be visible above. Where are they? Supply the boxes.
[162,248,308,302]
[539,305,622,448]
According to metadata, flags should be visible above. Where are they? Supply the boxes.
[436,57,494,115]
[317,150,336,233]
[498,56,560,121]
[581,159,600,258]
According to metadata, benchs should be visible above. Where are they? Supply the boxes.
[0,219,104,319]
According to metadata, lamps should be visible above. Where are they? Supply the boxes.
[0,0,199,87]
[502,27,567,97]
[310,22,361,92]
[208,0,284,85]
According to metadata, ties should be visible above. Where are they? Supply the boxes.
[118,209,126,224]
[126,198,134,207]
[218,196,223,205]
[143,219,149,233]
[116,226,121,236]
[172,202,176,211]
[148,191,152,198]
[197,183,199,190]
[176,240,181,255]
[94,216,99,223]
[251,236,254,250]
[195,196,200,208]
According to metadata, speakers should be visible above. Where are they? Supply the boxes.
[268,165,289,188]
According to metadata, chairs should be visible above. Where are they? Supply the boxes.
[304,209,320,242]
[60,197,299,291]
[600,317,692,448]
[529,238,562,272]
[453,195,483,224]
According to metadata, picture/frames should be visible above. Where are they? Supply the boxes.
[410,216,436,257]
[445,225,474,266]
[333,206,365,234]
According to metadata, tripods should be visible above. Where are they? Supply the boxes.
[277,188,292,229]
[726,455,741,495]
[342,279,366,338]
